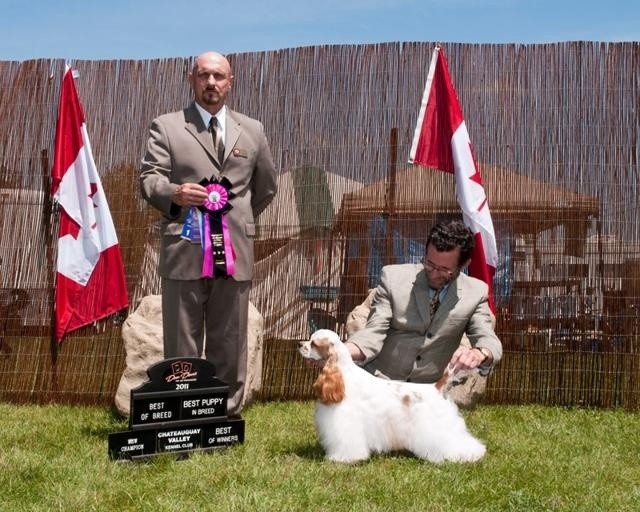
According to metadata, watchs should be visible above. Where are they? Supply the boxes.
[476,346,489,367]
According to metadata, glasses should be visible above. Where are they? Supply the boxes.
[420,253,458,276]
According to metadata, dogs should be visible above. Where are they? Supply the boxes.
[296,328,487,465]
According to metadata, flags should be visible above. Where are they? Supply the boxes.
[51,67,131,346]
[408,43,499,314]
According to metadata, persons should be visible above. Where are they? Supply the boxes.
[307,219,505,383]
[139,50,278,415]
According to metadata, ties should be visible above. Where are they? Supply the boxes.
[430,288,442,315]
[209,117,225,166]
